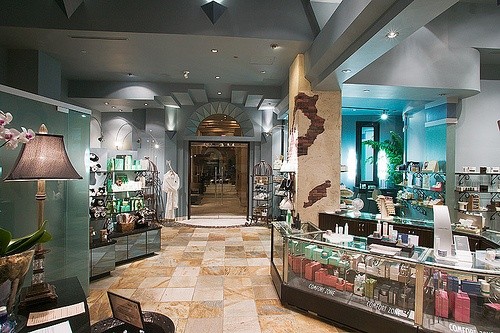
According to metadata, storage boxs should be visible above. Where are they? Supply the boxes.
[402,172,480,212]
[287,241,500,325]
[106,172,142,192]
[107,155,149,171]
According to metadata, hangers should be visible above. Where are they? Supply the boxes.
[170,168,175,177]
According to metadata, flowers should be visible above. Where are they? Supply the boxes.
[0,109,36,150]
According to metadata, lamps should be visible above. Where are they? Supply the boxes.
[381,110,388,121]
[3,133,84,308]
[280,162,296,181]
[123,129,160,148]
[116,122,141,143]
[92,116,104,141]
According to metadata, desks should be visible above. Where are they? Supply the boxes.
[6,277,175,333]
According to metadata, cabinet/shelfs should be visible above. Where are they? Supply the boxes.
[89,169,162,282]
[394,170,500,220]
[418,249,500,333]
[281,231,428,333]
[319,213,500,252]
[245,159,288,227]
[271,220,320,333]
